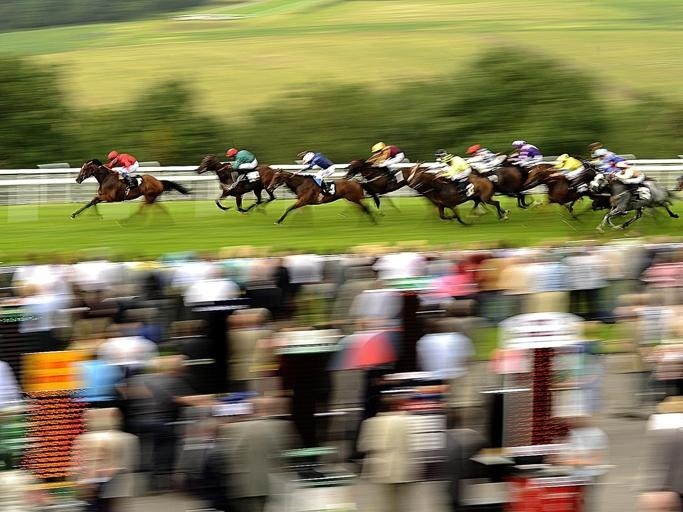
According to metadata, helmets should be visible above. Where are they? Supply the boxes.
[468,145,480,152]
[226,149,237,156]
[108,151,118,161]
[303,152,315,165]
[512,141,526,148]
[435,149,447,157]
[371,142,385,152]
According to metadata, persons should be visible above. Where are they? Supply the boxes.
[433,150,475,195]
[298,152,336,196]
[551,153,585,189]
[104,151,140,189]
[612,161,646,194]
[464,145,499,184]
[589,149,627,191]
[370,142,404,182]
[509,141,543,174]
[586,143,612,166]
[0,233,682,511]
[225,148,258,183]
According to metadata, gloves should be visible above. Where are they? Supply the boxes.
[226,165,233,171]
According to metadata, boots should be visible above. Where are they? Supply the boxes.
[125,175,136,189]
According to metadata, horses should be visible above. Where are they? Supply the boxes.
[68,157,195,230]
[266,167,378,227]
[492,159,681,236]
[342,157,422,215]
[406,161,510,225]
[194,153,276,213]
